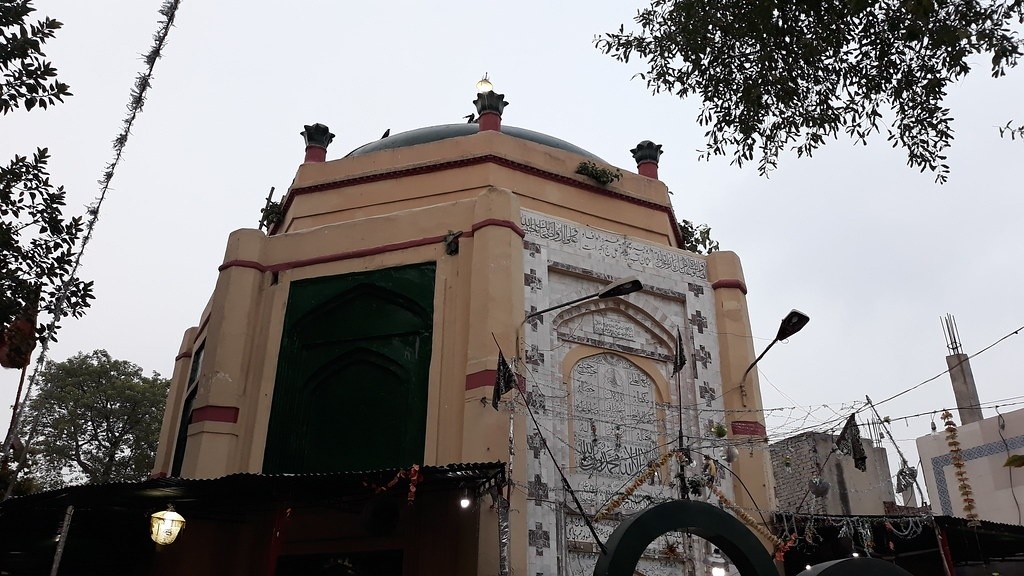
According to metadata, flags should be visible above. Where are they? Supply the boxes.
[831,412,868,473]
[491,350,519,411]
[0,284,43,369]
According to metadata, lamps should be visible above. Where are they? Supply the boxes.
[149,506,188,547]
[476,72,493,92]
[739,311,810,407]
[517,274,643,359]
